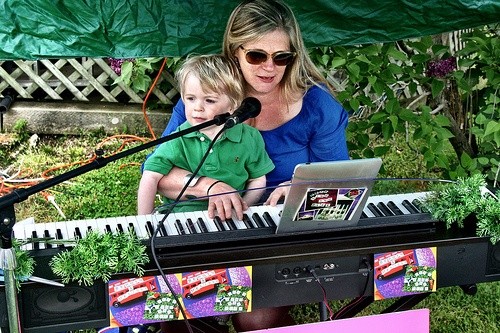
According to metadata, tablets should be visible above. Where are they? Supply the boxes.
[272,158,383,232]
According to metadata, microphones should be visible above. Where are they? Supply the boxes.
[151,95,262,333]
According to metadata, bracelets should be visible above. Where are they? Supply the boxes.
[206,180,223,196]
[183,173,206,199]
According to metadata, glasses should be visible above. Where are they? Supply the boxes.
[239,44,298,66]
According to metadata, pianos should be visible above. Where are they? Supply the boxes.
[0,186,492,332]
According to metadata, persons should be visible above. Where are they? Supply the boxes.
[129,52,276,333]
[141,0,354,333]
